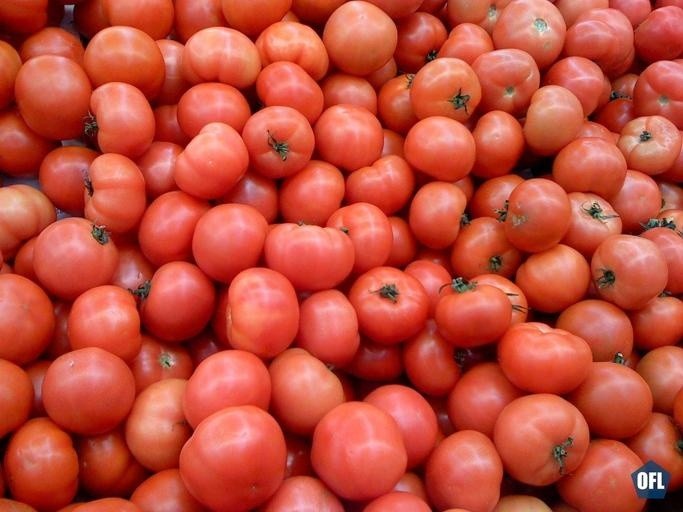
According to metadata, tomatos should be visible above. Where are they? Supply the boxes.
[0,1,682,510]
